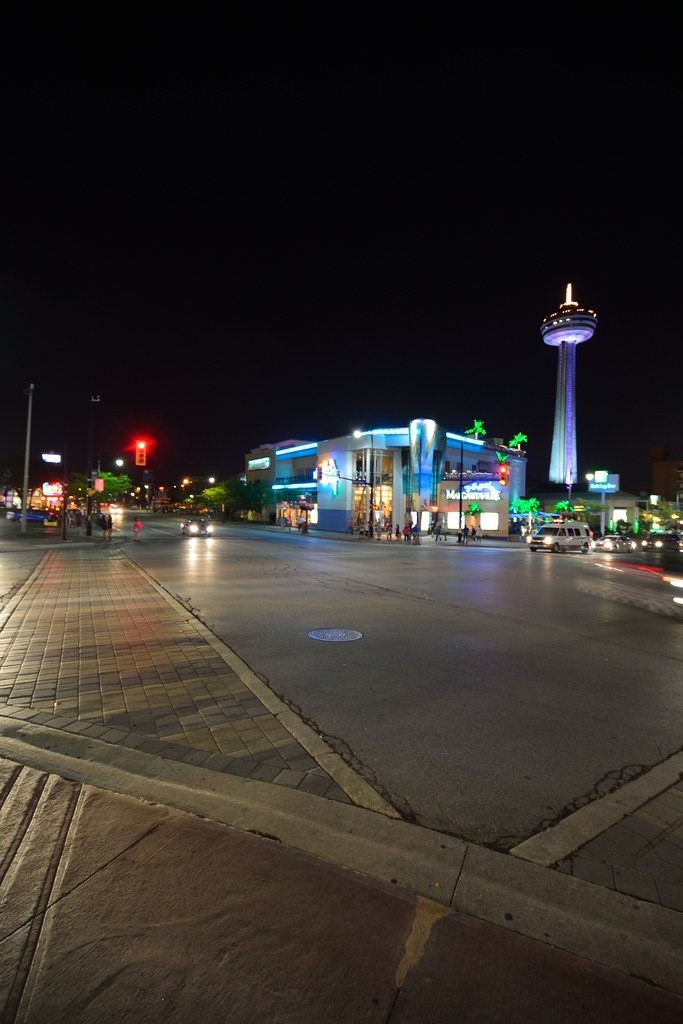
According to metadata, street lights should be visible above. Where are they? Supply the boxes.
[354,427,375,535]
[41,448,68,541]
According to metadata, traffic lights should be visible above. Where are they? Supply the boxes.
[499,464,508,485]
[136,439,146,466]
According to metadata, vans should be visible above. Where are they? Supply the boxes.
[526,522,592,554]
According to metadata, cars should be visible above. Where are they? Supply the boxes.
[592,534,637,553]
[181,518,211,537]
[643,532,680,552]
[6,509,50,523]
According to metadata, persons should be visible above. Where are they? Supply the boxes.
[47,503,145,542]
[277,514,305,532]
[346,513,485,546]
